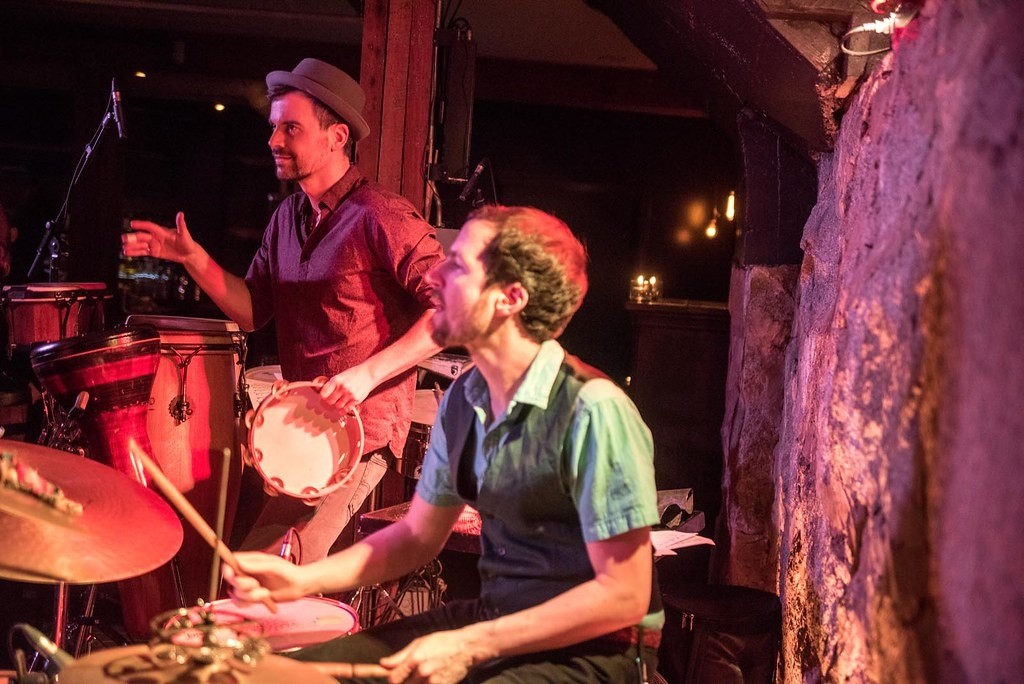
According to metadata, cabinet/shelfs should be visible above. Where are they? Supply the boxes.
[630,321,731,503]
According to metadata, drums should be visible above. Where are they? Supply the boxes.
[0,278,367,684]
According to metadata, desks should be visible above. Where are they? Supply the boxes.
[351,501,706,624]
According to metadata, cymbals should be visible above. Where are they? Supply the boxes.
[241,375,367,507]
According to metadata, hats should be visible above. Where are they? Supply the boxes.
[267,58,370,141]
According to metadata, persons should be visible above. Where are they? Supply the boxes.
[0,189,28,293]
[122,57,447,598]
[216,201,665,684]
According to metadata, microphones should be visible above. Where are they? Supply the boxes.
[112,78,128,139]
[460,159,486,201]
[280,529,294,562]
